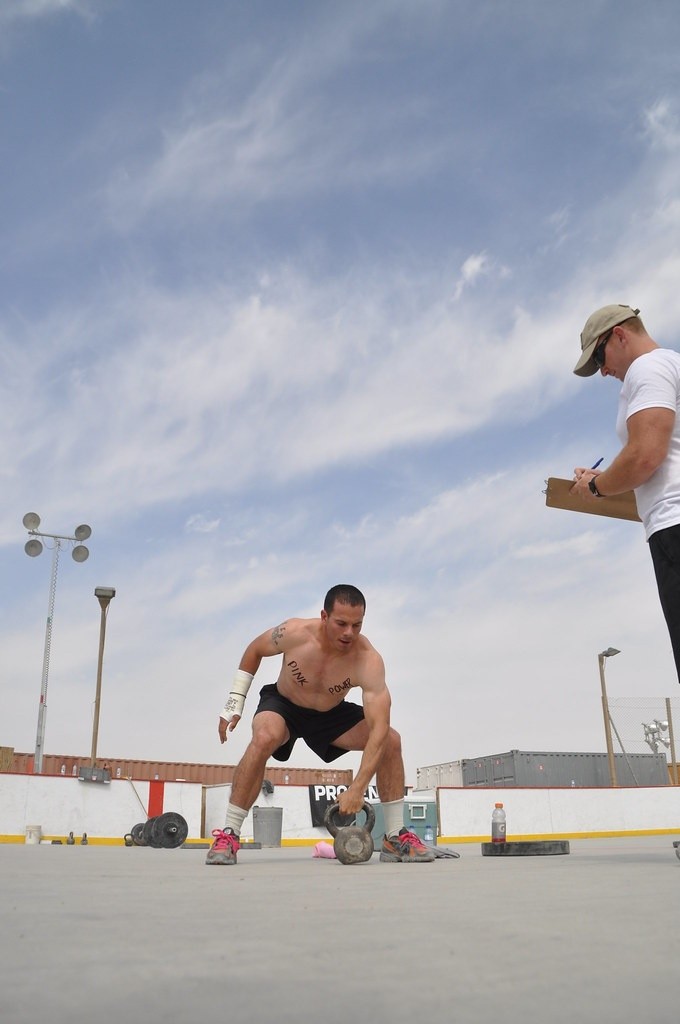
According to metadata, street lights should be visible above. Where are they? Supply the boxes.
[598,647,621,786]
[22,512,92,772]
[87,585,116,770]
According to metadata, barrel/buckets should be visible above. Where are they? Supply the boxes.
[25,825,41,844]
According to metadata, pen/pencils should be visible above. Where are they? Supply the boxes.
[590,457,604,470]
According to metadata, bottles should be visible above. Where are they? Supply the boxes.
[154,773,159,779]
[409,826,416,834]
[72,764,78,775]
[285,774,289,784]
[424,826,434,845]
[116,767,121,778]
[492,803,507,842]
[61,764,66,774]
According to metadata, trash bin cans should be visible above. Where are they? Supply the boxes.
[252,804,283,848]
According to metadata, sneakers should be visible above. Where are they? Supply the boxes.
[206,826,240,865]
[379,825,434,862]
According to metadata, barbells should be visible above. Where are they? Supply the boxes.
[131,822,147,847]
[142,812,189,849]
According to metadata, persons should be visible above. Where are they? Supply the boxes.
[569,304,680,862]
[204,584,436,865]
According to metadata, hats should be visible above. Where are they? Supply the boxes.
[573,304,641,378]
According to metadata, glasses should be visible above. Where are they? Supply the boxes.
[593,329,614,368]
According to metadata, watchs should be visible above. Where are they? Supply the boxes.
[588,474,606,497]
